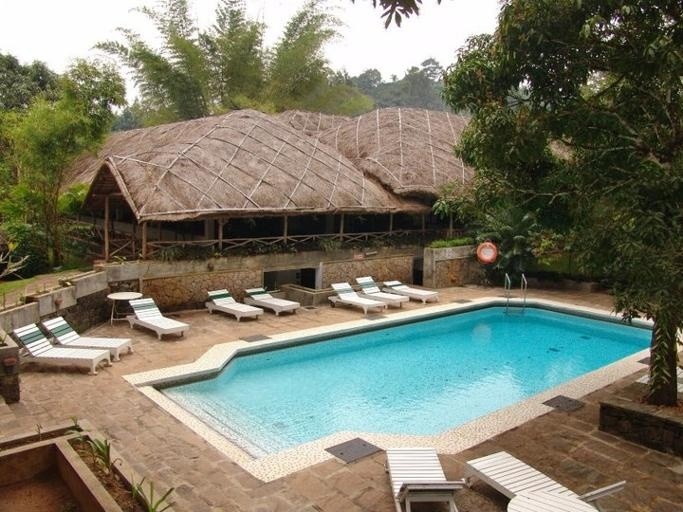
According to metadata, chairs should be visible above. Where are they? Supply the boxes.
[42,315,133,362]
[124,297,190,341]
[13,322,113,376]
[383,445,465,511]
[243,287,300,317]
[461,449,627,501]
[203,288,263,322]
[327,276,440,315]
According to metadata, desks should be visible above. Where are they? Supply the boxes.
[505,491,598,512]
[105,291,143,329]
